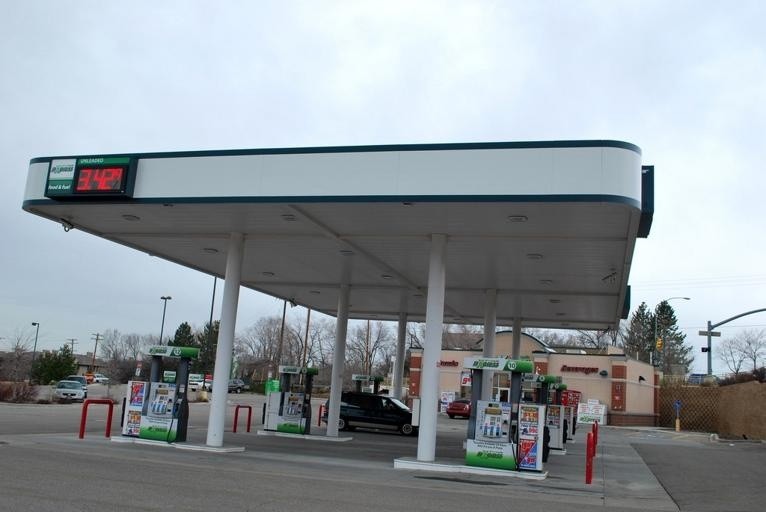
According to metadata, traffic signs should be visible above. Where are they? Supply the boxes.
[698,331,722,337]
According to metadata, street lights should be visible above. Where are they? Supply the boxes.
[158,295,172,347]
[654,296,691,363]
[27,321,39,385]
[700,346,712,377]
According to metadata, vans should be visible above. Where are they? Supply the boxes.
[685,373,721,386]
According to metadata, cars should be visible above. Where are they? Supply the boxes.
[181,373,247,396]
[445,397,471,420]
[45,369,112,405]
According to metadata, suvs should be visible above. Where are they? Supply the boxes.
[320,389,419,438]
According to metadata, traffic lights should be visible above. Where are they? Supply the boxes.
[656,338,662,352]
